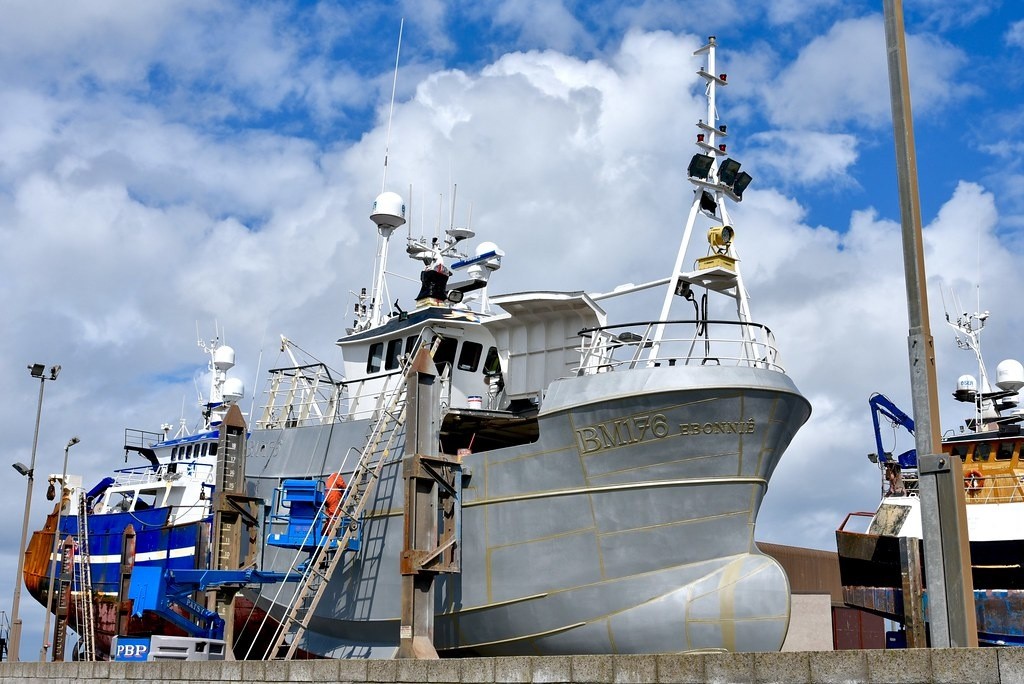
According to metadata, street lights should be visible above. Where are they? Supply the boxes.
[7,363,62,661]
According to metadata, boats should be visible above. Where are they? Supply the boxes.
[22,18,813,660]
[835,285,1024,646]
